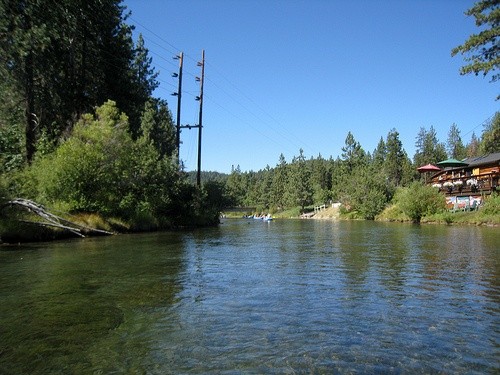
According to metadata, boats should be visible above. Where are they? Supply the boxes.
[247,215,273,221]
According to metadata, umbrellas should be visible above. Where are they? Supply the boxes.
[417,163,441,171]
[436,157,465,166]
